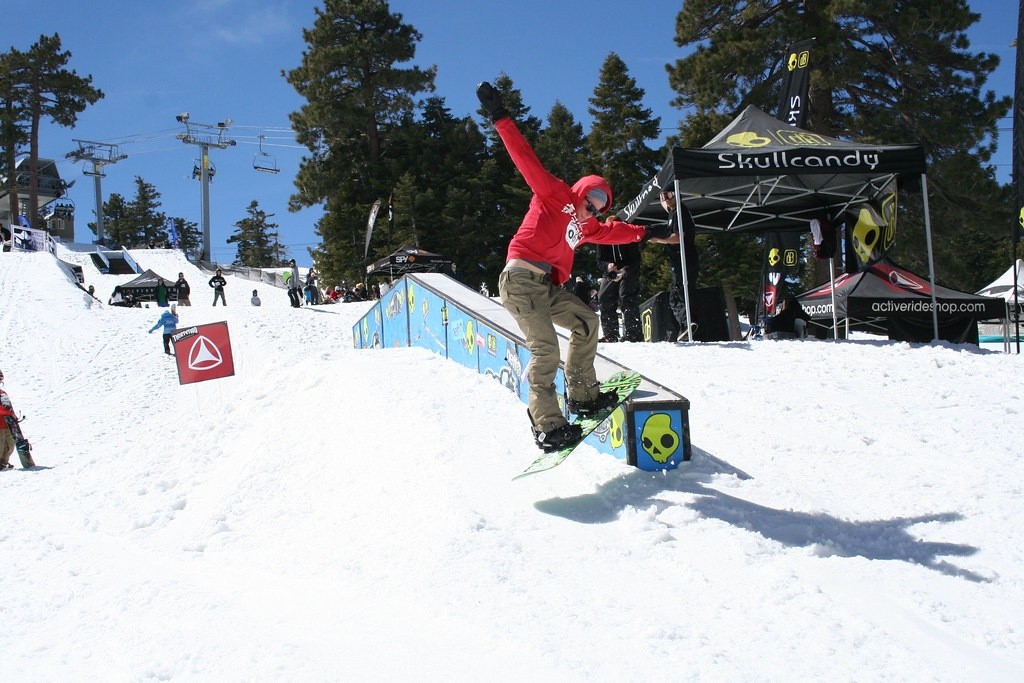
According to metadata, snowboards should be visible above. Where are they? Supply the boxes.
[510,369,642,481]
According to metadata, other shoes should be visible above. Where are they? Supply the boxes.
[536,424,584,449]
[678,322,698,342]
[569,387,619,416]
[0,462,14,470]
[597,333,619,343]
[619,332,644,343]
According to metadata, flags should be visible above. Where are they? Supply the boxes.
[363,187,394,258]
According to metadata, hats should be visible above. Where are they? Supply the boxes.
[661,181,675,192]
[587,187,608,207]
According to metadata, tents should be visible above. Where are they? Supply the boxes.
[975,258,1024,356]
[120,269,179,302]
[1011,0,1024,355]
[596,105,944,349]
[787,254,1006,348]
[366,242,452,287]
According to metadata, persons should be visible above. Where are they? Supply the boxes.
[174,272,192,306]
[647,184,699,342]
[251,289,261,305]
[153,278,170,307]
[597,262,622,342]
[109,286,134,307]
[0,369,33,472]
[208,268,228,306]
[0,222,11,244]
[148,304,182,355]
[89,285,94,295]
[477,82,674,450]
[609,263,648,340]
[286,258,380,307]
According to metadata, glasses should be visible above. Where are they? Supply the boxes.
[584,197,602,218]
[660,191,676,200]
